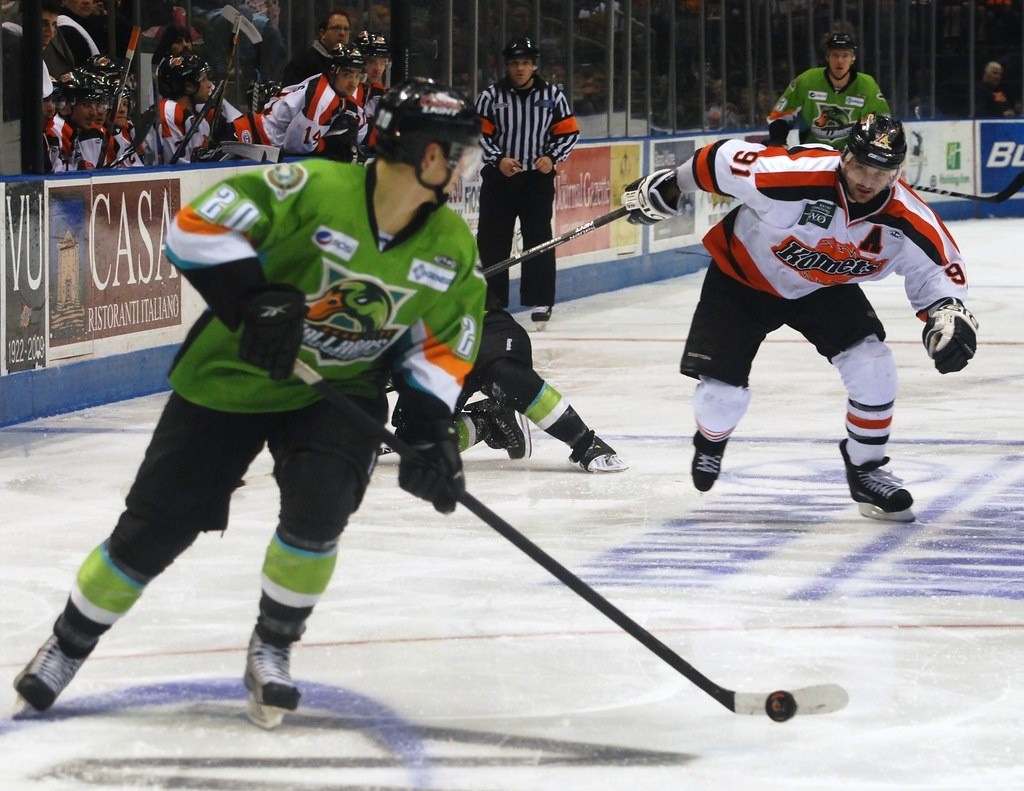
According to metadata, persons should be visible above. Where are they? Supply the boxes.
[620,113,978,522]
[764,33,892,149]
[390,279,630,472]
[0,0,1024,174]
[12,76,488,729]
[474,36,581,331]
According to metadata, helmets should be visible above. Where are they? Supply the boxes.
[155,53,212,100]
[353,31,392,58]
[40,55,133,114]
[842,113,908,173]
[825,33,858,64]
[328,43,367,78]
[372,77,482,170]
[502,36,540,65]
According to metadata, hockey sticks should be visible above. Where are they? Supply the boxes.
[95,23,141,168]
[207,141,267,164]
[295,358,850,717]
[105,109,158,168]
[482,208,626,276]
[211,4,265,137]
[910,171,1024,205]
[325,127,350,137]
[253,143,285,164]
[168,79,225,165]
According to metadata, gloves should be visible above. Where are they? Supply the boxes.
[768,119,793,148]
[323,115,359,162]
[394,416,466,514]
[235,282,308,382]
[624,167,681,226]
[924,302,980,375]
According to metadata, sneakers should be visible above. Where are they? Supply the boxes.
[245,625,300,729]
[466,398,532,460]
[569,431,629,474]
[10,632,84,719]
[840,438,915,523]
[690,430,731,491]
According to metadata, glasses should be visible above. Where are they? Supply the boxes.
[322,26,350,34]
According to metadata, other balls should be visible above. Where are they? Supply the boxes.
[766,691,798,723]
